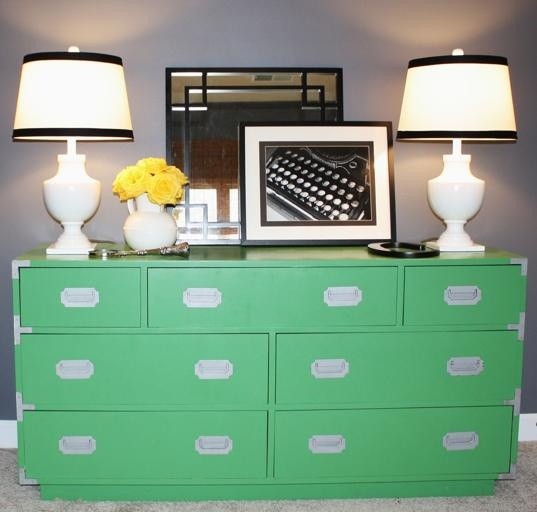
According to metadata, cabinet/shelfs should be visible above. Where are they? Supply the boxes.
[9,245,528,501]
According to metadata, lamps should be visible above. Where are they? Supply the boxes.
[394,47,518,254]
[11,46,134,255]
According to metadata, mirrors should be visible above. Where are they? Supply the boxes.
[163,65,343,248]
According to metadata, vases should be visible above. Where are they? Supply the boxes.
[123,193,177,251]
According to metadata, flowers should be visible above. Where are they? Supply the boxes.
[110,156,189,208]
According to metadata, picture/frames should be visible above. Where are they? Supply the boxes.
[236,120,397,248]
